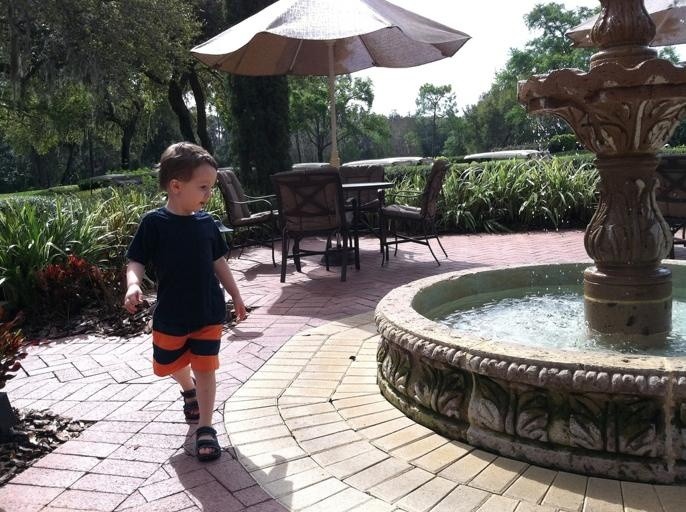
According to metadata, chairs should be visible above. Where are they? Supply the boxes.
[217,159,450,283]
[654,154,686,259]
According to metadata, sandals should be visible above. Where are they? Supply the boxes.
[196,426,221,460]
[180,378,200,420]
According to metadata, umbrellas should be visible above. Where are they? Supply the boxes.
[180,0,474,164]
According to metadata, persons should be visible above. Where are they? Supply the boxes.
[117,140,246,462]
